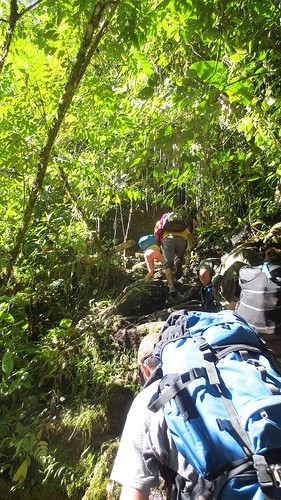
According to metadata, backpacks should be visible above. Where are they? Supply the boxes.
[138,234,156,251]
[144,307,281,500]
[161,210,187,232]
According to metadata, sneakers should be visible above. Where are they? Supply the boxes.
[169,287,175,297]
[174,258,184,280]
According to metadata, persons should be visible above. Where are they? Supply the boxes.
[109,333,199,500]
[145,244,165,279]
[200,249,237,310]
[154,212,194,297]
